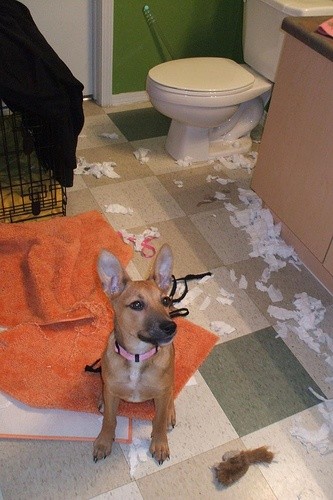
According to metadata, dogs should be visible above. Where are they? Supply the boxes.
[92,243,178,466]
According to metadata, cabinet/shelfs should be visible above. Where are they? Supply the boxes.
[249,35,333,299]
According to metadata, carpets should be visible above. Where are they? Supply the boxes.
[0,208,219,444]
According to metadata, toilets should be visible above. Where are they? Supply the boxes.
[146,0,333,164]
